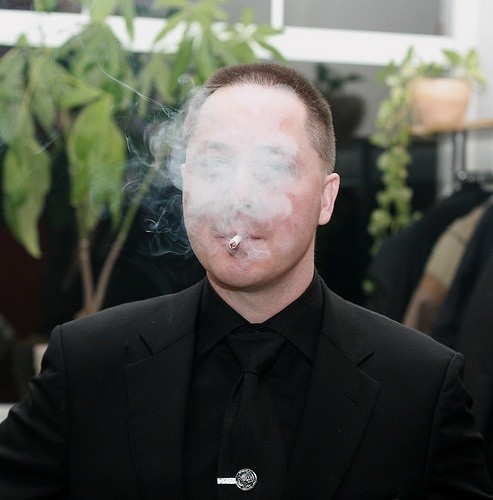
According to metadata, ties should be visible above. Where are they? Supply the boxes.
[212,328,287,500]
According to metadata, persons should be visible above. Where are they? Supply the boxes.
[0,64,492,500]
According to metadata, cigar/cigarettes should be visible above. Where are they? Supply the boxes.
[225,234,251,251]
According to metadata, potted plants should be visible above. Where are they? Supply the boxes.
[367,40,481,251]
